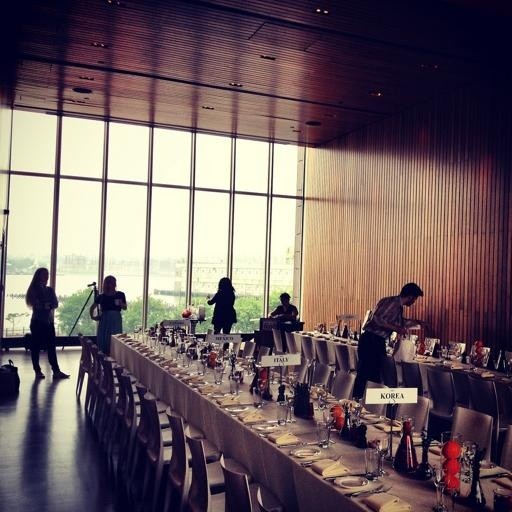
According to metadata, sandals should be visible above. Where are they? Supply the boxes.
[52,372,71,380]
[36,371,46,378]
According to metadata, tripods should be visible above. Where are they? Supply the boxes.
[62,286,100,351]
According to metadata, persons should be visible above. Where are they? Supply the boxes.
[270,293,298,322]
[352,283,424,419]
[26,268,69,379]
[207,278,237,351]
[90,276,127,356]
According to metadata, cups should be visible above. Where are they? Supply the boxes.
[45,302,51,309]
[198,305,205,320]
[227,367,511,512]
[126,324,256,399]
[114,299,120,305]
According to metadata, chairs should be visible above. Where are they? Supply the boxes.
[73,321,511,512]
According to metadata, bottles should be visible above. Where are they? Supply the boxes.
[318,322,359,341]
[416,338,512,381]
